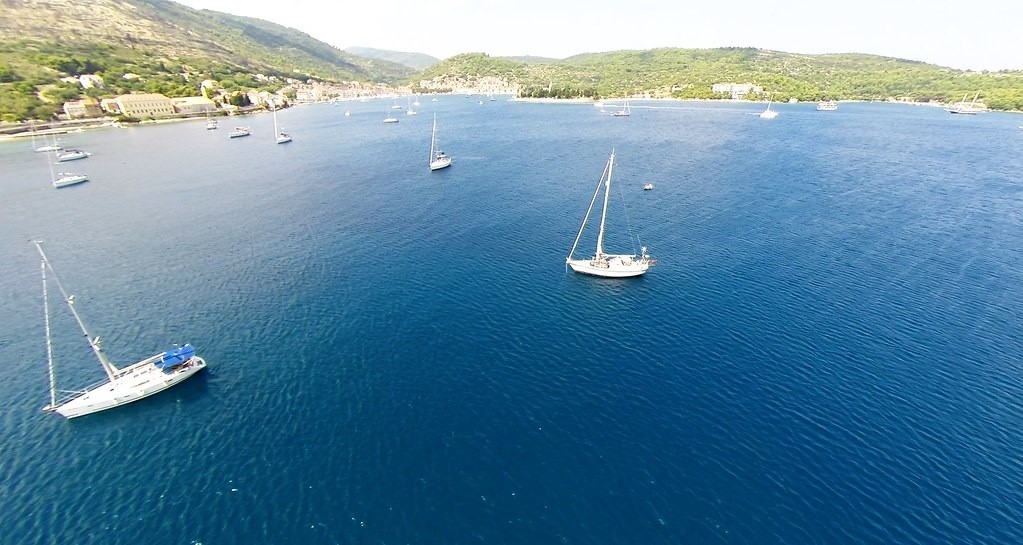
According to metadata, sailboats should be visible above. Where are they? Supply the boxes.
[392,90,402,109]
[383,101,399,123]
[43,131,88,188]
[51,117,92,162]
[307,87,400,106]
[28,237,209,421]
[412,95,421,106]
[614,92,631,116]
[272,99,292,144]
[428,112,451,171]
[205,105,218,130]
[759,94,779,118]
[564,147,659,279]
[31,120,62,153]
[407,93,417,116]
[943,92,980,114]
[465,88,497,105]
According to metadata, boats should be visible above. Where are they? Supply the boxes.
[816,100,838,111]
[432,98,438,102]
[230,127,251,138]
[67,128,87,133]
